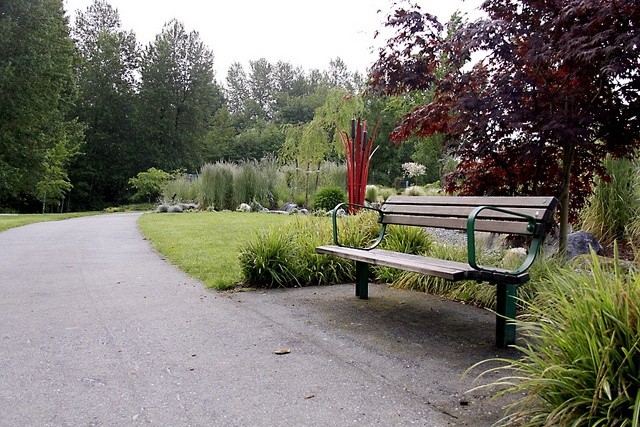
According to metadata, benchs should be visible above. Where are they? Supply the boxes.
[314,194,555,351]
